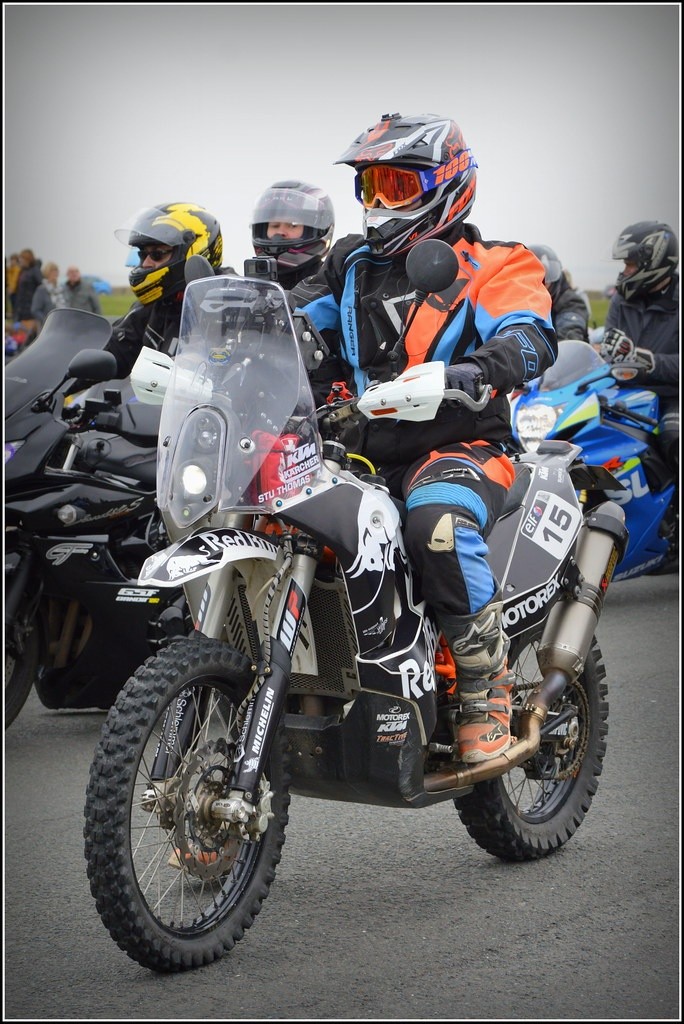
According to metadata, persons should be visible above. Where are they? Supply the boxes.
[244,179,336,392]
[603,218,679,490]
[285,108,560,765]
[527,241,590,340]
[7,248,101,322]
[63,200,242,422]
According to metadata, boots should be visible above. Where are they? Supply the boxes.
[438,573,517,764]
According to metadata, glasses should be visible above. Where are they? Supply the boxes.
[137,249,172,261]
[360,165,424,210]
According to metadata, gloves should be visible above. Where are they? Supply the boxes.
[444,362,483,408]
[599,328,656,376]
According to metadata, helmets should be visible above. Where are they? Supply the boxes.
[332,110,478,259]
[611,221,680,302]
[249,180,336,276]
[527,243,562,304]
[113,201,223,308]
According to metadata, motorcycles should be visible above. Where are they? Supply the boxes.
[5,306,218,731]
[508,339,680,586]
[79,236,632,971]
